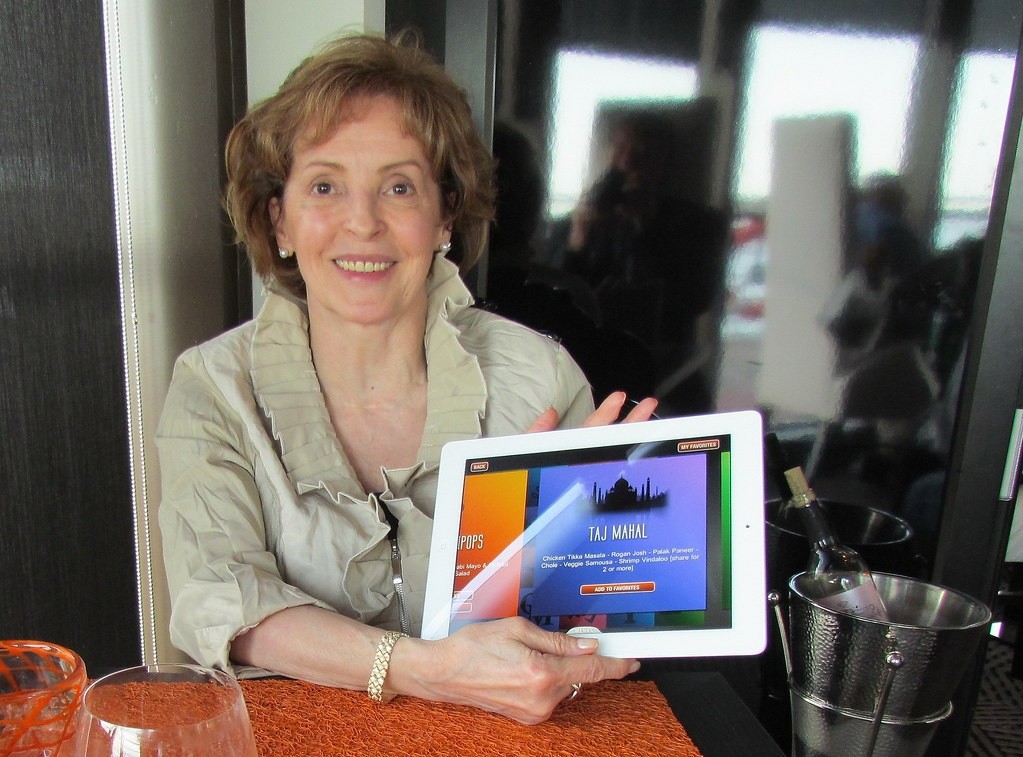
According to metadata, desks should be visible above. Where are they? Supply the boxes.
[0,658,782,757]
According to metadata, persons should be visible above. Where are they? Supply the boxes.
[572,114,718,393]
[854,182,933,372]
[152,34,662,723]
[484,122,536,308]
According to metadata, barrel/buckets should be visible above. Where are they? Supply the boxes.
[790,571,992,757]
[762,498,915,704]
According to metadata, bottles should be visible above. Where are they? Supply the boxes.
[784,466,889,623]
[766,432,808,535]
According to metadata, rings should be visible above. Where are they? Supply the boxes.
[568,683,583,700]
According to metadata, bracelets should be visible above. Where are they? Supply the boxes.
[368,631,408,702]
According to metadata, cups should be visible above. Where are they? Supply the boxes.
[78,663,259,757]
[0,641,85,757]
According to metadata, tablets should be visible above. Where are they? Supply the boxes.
[420,409,769,660]
